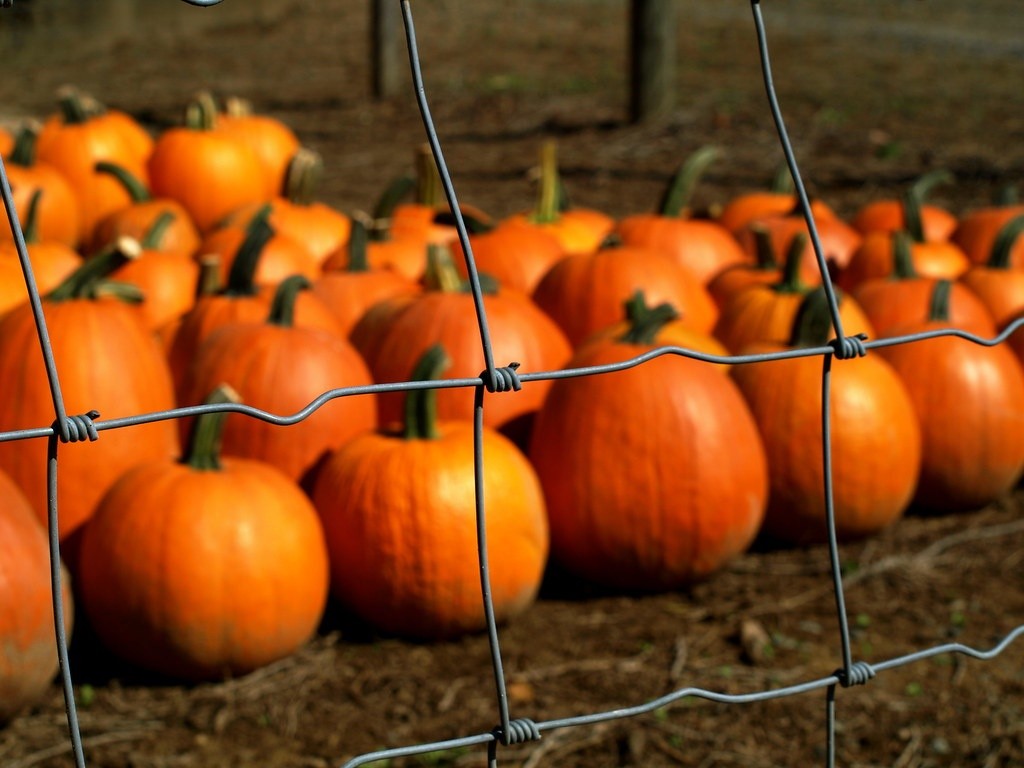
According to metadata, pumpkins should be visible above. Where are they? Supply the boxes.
[0,84,1024,718]
